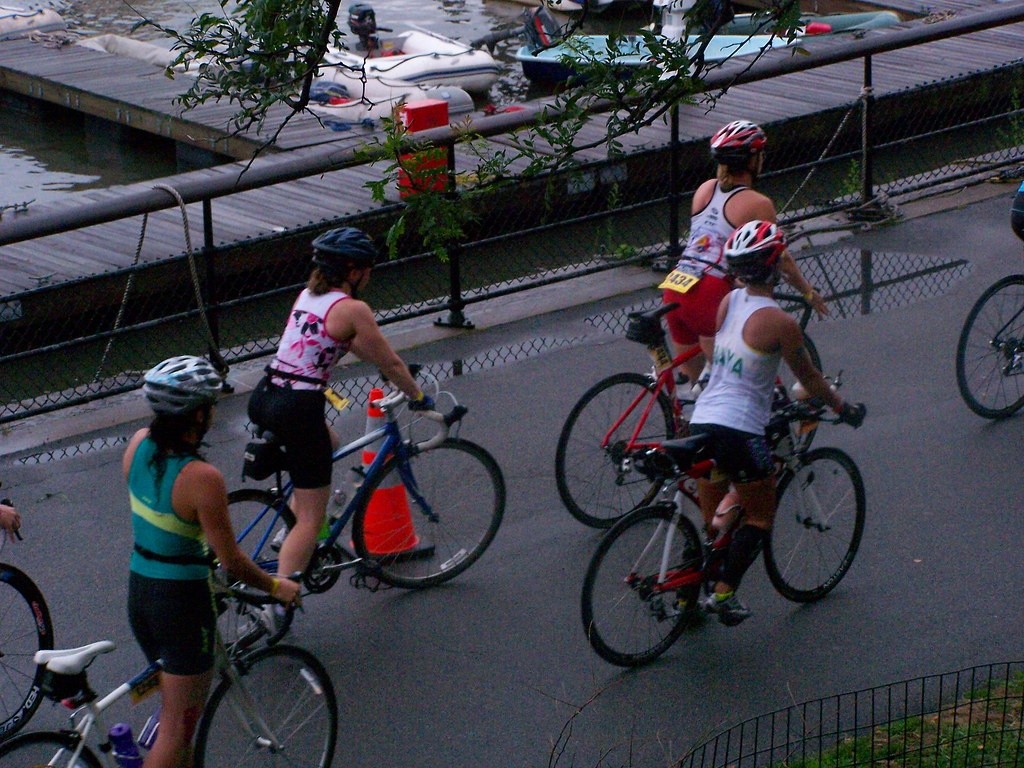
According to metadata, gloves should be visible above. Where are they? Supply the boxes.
[407,392,435,412]
[835,398,864,425]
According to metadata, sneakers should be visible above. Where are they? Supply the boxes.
[704,592,754,617]
[672,602,712,627]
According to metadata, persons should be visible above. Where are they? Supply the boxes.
[672,219,866,617]
[123,353,302,768]
[237,226,436,645]
[0,504,21,543]
[1010,180,1024,241]
[662,120,830,395]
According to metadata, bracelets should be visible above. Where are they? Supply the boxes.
[410,389,424,401]
[802,287,814,300]
[831,396,846,413]
[270,577,279,596]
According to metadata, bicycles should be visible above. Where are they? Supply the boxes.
[209,363,508,654]
[556,283,825,531]
[956,224,1024,419]
[0,550,337,768]
[580,392,866,669]
[0,498,54,741]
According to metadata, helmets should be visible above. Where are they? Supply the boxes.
[725,220,786,273]
[312,227,377,267]
[710,120,767,157]
[143,356,223,413]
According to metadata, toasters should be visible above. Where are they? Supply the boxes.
[712,482,744,531]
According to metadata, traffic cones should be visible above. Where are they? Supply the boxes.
[342,384,439,571]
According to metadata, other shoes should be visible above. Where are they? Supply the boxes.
[271,524,288,552]
[259,604,283,631]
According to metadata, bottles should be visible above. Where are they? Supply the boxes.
[675,371,695,420]
[136,702,164,750]
[109,722,144,768]
[690,361,711,396]
[314,465,366,548]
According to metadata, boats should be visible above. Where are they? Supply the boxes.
[515,5,902,92]
[208,14,504,134]
